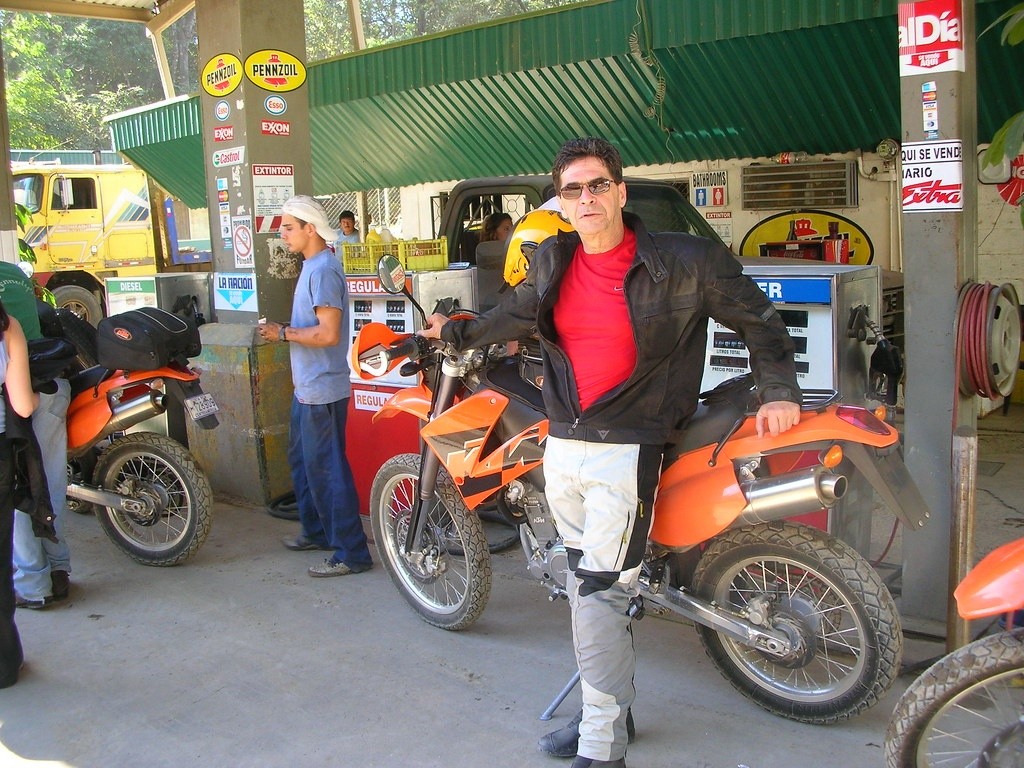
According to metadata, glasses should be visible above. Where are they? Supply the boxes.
[559,178,615,200]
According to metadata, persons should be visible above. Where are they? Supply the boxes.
[331,210,361,265]
[478,213,514,246]
[416,136,803,768]
[0,262,74,692]
[257,195,374,578]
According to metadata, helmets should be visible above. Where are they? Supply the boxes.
[502,209,577,288]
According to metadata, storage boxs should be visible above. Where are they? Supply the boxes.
[342,236,448,276]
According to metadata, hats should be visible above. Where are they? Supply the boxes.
[283,195,337,243]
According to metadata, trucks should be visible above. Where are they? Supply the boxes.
[11,165,212,328]
[429,176,734,355]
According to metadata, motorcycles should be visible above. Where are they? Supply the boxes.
[882,536,1024,768]
[348,254,930,727]
[67,363,219,568]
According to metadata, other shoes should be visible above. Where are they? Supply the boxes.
[539,708,638,768]
[284,534,330,552]
[14,571,72,609]
[309,558,353,578]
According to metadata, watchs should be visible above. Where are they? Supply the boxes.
[279,323,289,343]
